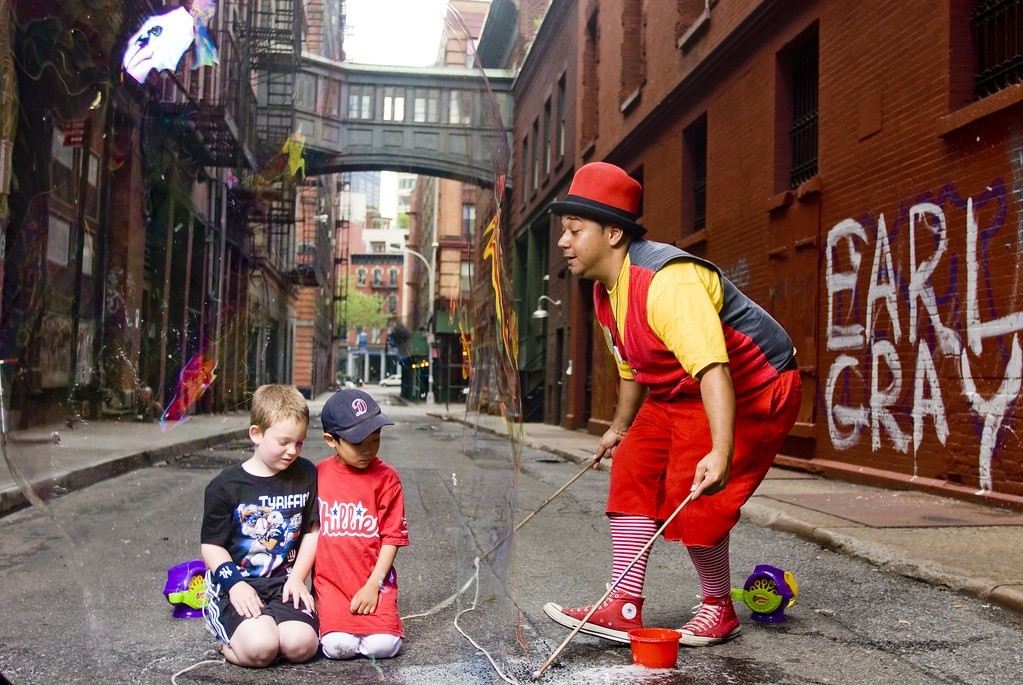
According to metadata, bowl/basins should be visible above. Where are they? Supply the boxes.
[628,628,682,669]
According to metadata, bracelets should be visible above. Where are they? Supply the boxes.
[214,561,244,591]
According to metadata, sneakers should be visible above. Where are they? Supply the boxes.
[673,594,743,646]
[543,583,646,643]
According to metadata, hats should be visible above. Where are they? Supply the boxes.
[321,388,395,444]
[549,161,648,241]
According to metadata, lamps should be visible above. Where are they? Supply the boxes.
[532,295,562,319]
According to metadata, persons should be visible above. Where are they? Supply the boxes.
[200,383,409,668]
[543,161,802,646]
